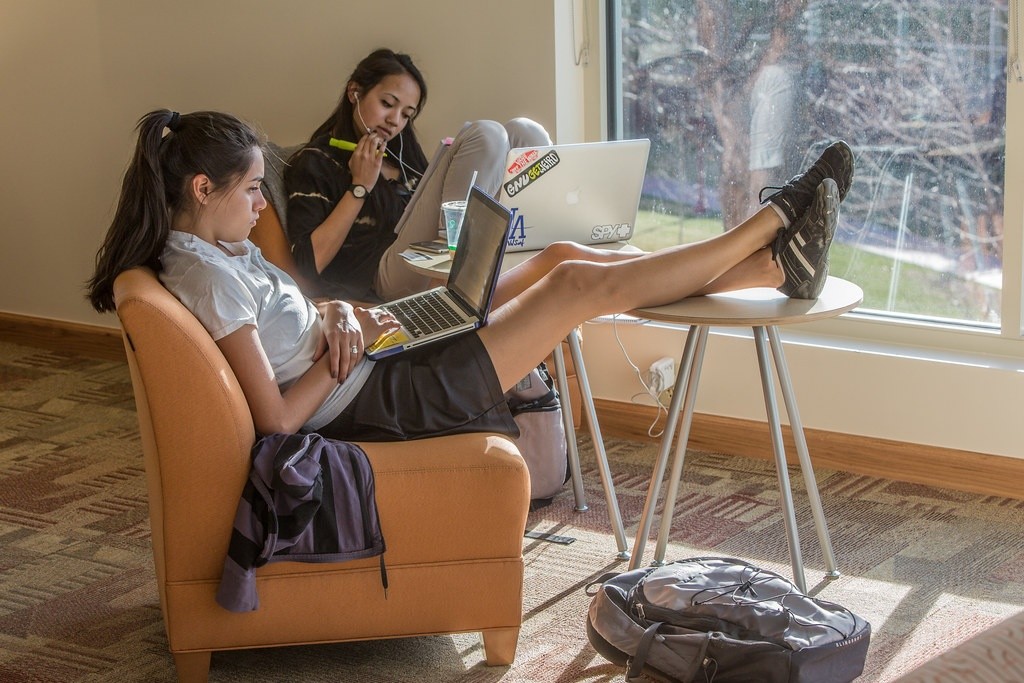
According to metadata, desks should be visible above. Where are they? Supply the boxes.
[398,224,648,554]
[613,274,865,598]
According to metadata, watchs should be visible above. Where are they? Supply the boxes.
[346,185,369,200]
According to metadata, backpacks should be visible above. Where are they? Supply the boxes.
[585,556,871,683]
[504,361,571,513]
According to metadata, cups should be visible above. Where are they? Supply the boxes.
[440,200,466,260]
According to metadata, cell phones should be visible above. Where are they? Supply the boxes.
[409,241,449,254]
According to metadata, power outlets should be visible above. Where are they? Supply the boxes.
[658,361,687,412]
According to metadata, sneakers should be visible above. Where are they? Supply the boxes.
[770,178,840,300]
[759,140,855,225]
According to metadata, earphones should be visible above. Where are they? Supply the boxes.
[354,92,358,99]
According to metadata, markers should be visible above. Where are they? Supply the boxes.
[329,138,387,157]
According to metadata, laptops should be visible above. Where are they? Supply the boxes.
[500,139,651,253]
[360,183,512,363]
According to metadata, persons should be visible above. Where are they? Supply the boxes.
[284,48,553,300]
[84,109,855,441]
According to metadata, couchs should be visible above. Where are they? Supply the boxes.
[112,262,532,683]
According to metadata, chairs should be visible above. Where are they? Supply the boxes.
[247,141,582,440]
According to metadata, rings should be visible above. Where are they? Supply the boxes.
[350,346,358,354]
